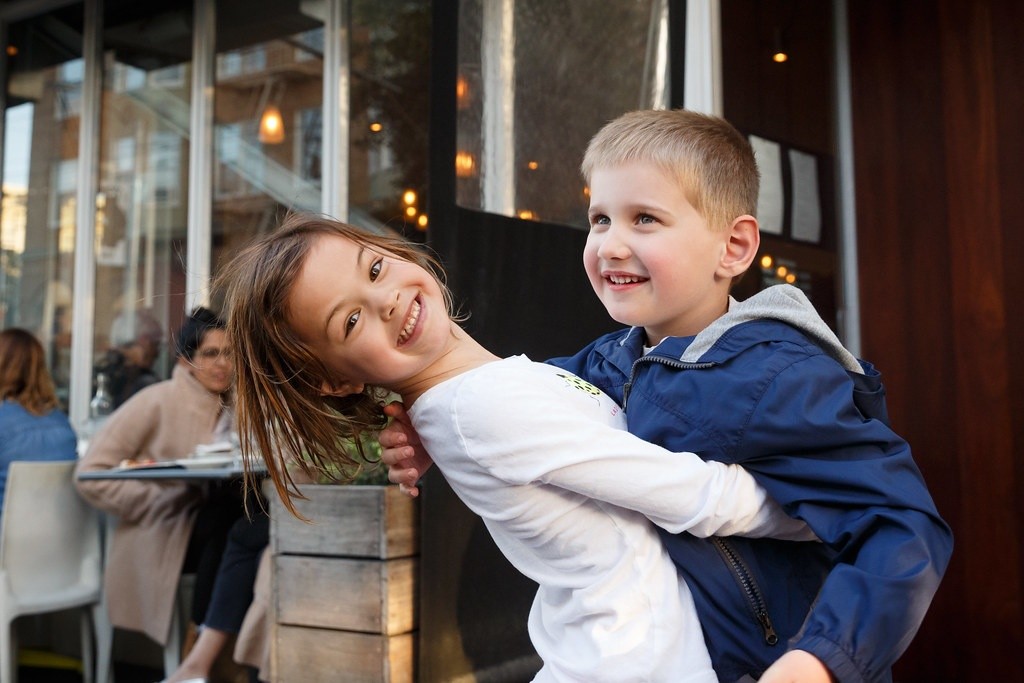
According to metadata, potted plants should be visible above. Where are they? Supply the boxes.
[265,416,414,683]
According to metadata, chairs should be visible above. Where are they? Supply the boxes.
[0,462,198,683]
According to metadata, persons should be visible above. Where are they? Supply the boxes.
[91,305,164,420]
[216,207,823,683]
[0,328,78,513]
[381,109,955,683]
[69,305,303,683]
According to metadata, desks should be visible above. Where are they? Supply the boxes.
[80,460,267,479]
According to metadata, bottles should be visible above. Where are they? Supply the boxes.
[91,372,113,421]
